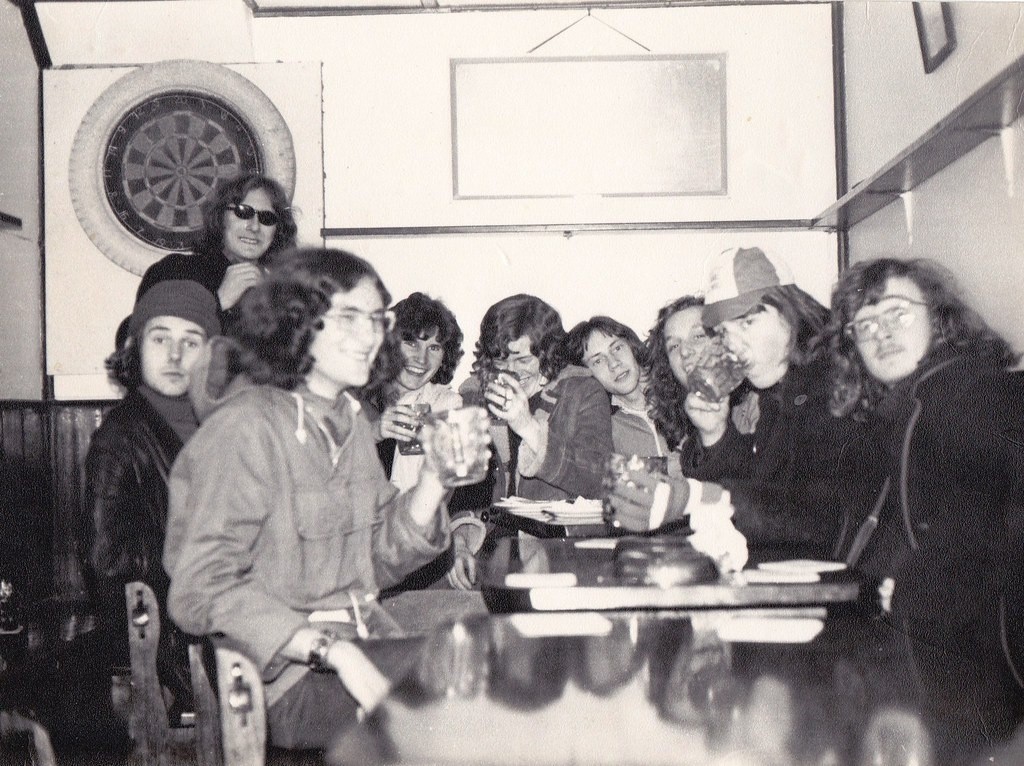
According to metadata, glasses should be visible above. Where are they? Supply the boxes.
[322,309,397,331]
[225,205,277,226]
[842,303,928,344]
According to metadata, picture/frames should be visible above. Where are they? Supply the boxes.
[452,53,728,199]
[911,1,957,74]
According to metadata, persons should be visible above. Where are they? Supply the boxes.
[459,292,612,501]
[603,298,761,533]
[679,245,1024,766]
[567,316,670,460]
[115,177,297,351]
[355,294,462,498]
[78,279,222,711]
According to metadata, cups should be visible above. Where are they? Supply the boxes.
[395,404,432,455]
[602,452,669,526]
[424,405,488,488]
[484,370,520,425]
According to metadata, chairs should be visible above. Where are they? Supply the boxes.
[81,571,267,766]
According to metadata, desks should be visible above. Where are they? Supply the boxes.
[332,497,1024,766]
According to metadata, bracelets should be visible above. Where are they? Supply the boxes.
[163,247,493,747]
[680,477,703,516]
[309,627,341,670]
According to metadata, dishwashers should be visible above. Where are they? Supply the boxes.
[687,333,755,404]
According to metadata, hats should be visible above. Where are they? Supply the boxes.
[701,247,795,328]
[130,279,220,339]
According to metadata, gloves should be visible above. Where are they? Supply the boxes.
[611,452,702,531]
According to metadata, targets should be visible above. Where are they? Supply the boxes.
[102,89,265,252]
